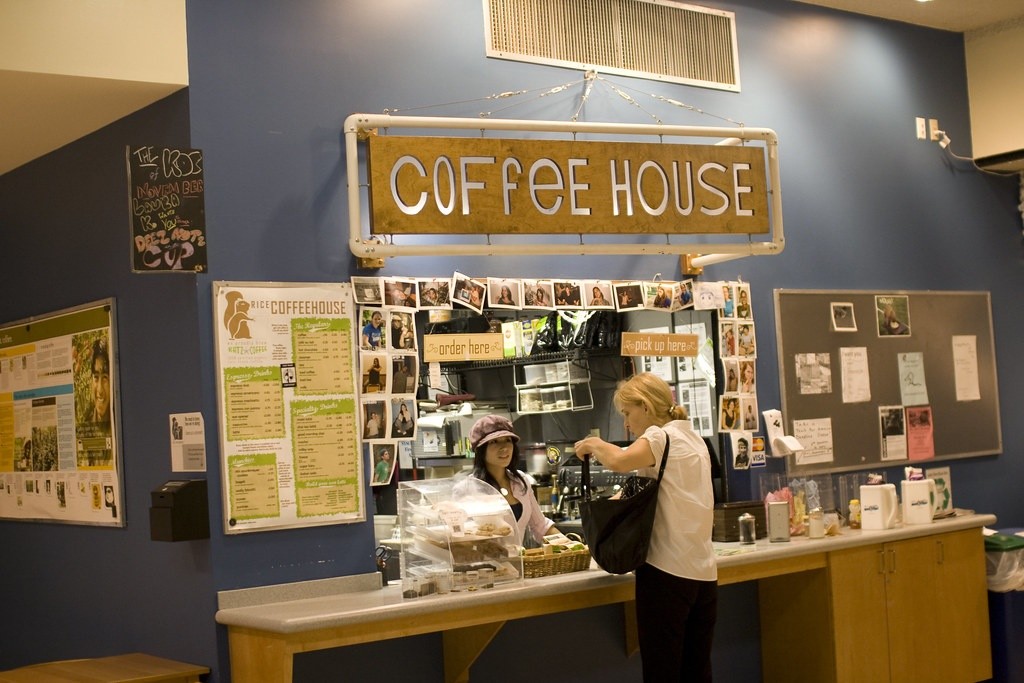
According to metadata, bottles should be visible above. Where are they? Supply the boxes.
[809,507,824,538]
[738,513,756,544]
[849,499,861,529]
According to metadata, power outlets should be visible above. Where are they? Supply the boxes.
[929,118,940,140]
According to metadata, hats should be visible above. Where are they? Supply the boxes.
[392,314,401,320]
[470,413,520,452]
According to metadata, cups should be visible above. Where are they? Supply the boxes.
[901,479,938,524]
[859,483,898,530]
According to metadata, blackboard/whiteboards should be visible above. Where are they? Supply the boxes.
[772,288,1004,479]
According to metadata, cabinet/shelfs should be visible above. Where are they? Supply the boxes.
[758,524,996,683]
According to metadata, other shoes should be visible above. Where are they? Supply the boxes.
[397,429,402,433]
[403,431,407,435]
[362,391,366,393]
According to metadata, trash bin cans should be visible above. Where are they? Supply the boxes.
[983,527,1024,683]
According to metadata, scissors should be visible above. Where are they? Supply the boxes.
[375,546,393,570]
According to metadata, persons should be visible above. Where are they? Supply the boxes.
[451,413,568,550]
[354,276,756,482]
[75,337,113,466]
[573,374,719,683]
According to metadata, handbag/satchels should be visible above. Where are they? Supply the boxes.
[578,428,669,575]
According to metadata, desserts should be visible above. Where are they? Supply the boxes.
[403,519,585,598]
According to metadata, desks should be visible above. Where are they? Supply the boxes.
[0,650,212,683]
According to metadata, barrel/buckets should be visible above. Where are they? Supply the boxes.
[374,515,398,558]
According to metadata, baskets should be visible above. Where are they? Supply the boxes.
[501,529,592,579]
